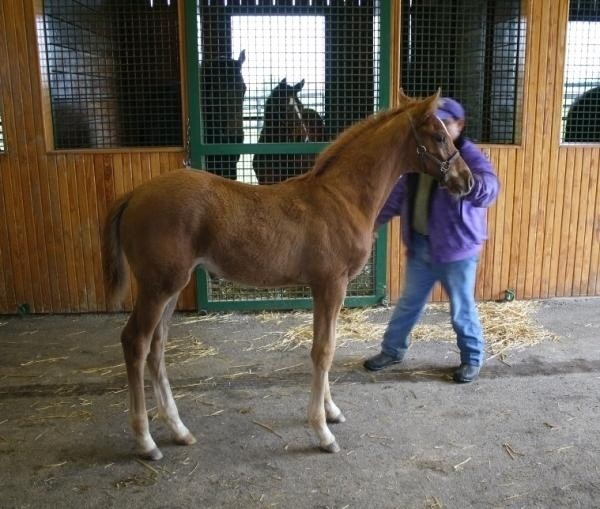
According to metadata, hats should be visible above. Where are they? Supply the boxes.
[435,97,466,120]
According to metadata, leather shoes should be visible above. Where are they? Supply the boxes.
[363,352,402,371]
[453,364,480,382]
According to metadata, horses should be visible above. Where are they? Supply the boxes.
[99,86,475,460]
[165,50,247,180]
[253,77,326,185]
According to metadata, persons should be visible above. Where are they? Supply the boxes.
[362,96,501,383]
